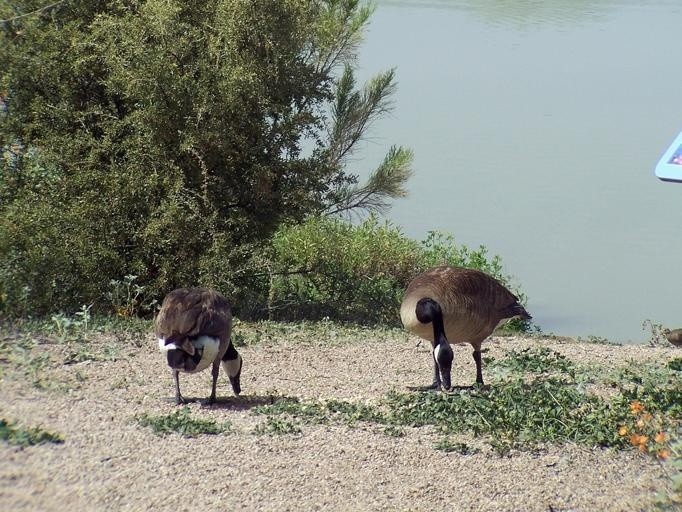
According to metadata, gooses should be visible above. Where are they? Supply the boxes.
[156,286,244,404]
[400,265,532,397]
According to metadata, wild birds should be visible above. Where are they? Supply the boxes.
[660,328,682,349]
